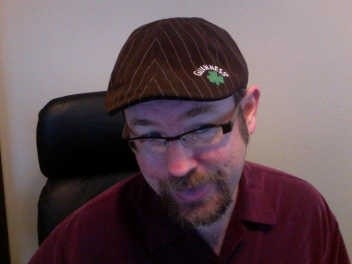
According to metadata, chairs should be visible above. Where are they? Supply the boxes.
[36,90,142,248]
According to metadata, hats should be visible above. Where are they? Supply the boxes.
[103,16,250,113]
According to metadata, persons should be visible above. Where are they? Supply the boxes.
[29,17,350,264]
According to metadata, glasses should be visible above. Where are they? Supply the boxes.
[123,98,242,160]
